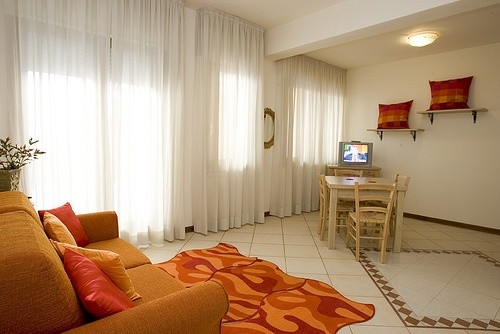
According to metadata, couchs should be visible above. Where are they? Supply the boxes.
[0,191,230,334]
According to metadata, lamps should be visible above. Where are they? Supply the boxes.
[406,32,439,47]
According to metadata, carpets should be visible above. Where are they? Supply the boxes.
[152,242,375,334]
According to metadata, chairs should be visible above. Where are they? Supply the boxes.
[316,169,411,264]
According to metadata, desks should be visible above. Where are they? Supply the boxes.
[325,176,408,253]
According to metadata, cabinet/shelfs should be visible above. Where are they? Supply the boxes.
[366,107,488,141]
[326,165,382,208]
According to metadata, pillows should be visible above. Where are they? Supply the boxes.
[38,202,142,319]
[426,76,473,111]
[377,100,413,129]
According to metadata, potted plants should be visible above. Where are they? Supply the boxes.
[0,136,47,191]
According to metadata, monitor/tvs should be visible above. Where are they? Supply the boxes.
[338,142,373,168]
